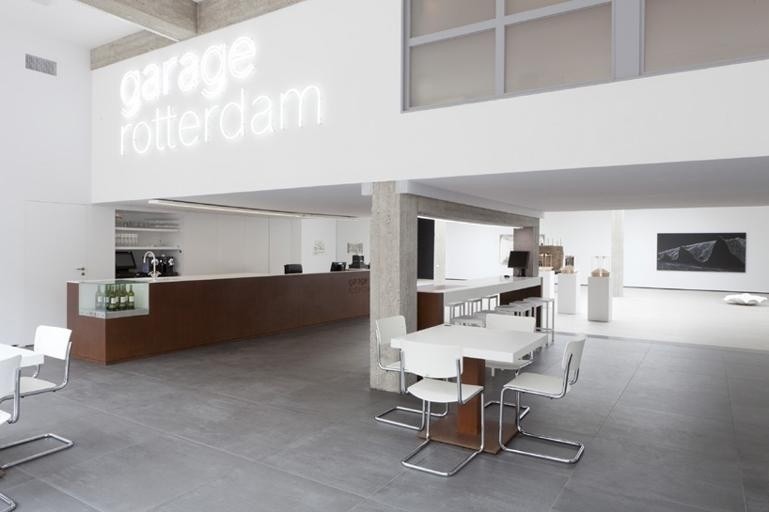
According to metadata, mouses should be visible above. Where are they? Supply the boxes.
[504,275,510,277]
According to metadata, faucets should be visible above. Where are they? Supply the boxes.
[142,251,162,279]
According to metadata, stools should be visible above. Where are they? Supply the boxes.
[445,295,554,364]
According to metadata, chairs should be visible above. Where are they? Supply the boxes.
[374,316,449,431]
[1,325,76,469]
[482,313,537,422]
[1,355,23,512]
[400,346,484,478]
[500,336,586,464]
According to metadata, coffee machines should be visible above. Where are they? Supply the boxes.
[147,256,178,278]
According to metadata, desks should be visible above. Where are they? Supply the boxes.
[391,324,548,454]
[1,345,46,479]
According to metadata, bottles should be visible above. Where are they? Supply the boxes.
[116,230,140,247]
[116,216,180,228]
[93,284,136,312]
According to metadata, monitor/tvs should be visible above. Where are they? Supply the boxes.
[507,251,530,277]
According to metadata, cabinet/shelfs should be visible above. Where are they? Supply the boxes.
[115,205,181,280]
